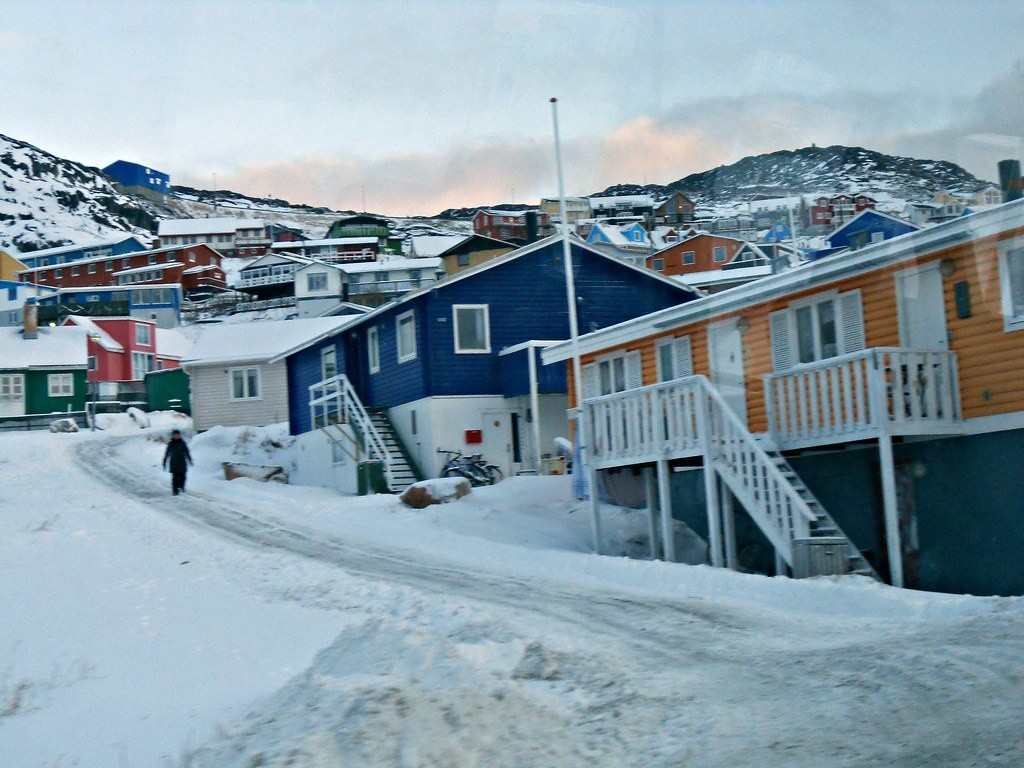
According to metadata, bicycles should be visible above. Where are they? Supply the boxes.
[436,447,504,488]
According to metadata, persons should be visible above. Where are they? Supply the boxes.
[162,430,193,497]
